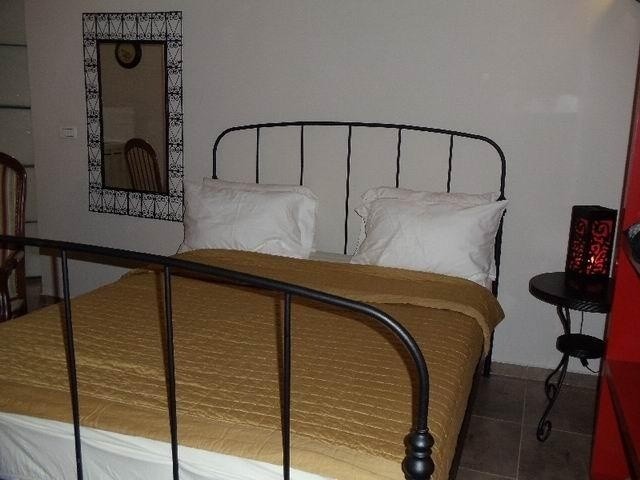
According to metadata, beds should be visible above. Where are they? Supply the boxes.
[1,121,508,480]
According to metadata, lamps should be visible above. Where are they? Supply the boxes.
[565,204,617,281]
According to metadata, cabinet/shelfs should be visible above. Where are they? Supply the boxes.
[588,42,640,479]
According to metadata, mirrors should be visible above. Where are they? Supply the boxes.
[83,10,185,223]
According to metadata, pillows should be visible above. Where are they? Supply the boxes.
[174,175,318,260]
[349,188,507,283]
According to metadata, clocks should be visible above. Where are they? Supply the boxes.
[115,40,142,69]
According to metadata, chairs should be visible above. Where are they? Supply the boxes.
[1,151,28,321]
[124,137,162,194]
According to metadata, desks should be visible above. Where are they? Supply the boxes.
[528,271,614,443]
